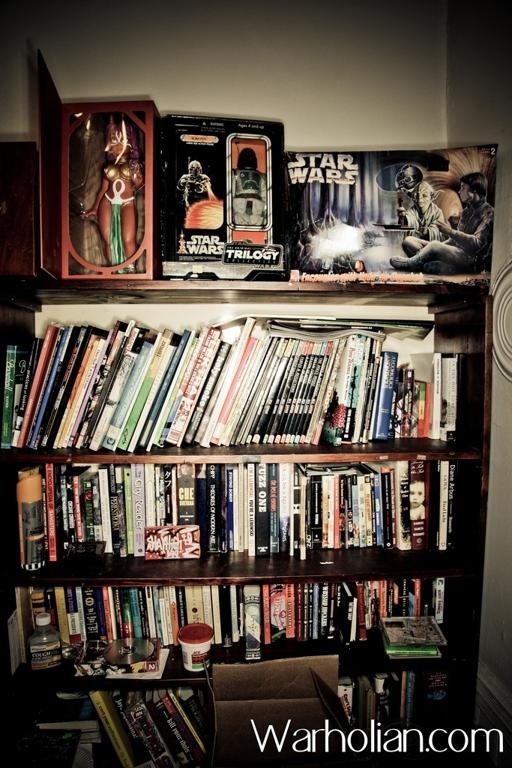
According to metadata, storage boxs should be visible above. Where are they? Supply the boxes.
[203,654,358,767]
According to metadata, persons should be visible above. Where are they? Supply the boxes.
[54,97,495,282]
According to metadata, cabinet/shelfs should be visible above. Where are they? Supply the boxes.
[0,270,495,768]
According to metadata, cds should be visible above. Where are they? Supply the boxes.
[102,637,155,666]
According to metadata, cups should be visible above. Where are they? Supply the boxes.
[178,623,215,672]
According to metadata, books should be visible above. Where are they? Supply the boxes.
[0,316,468,765]
[54,97,494,282]
